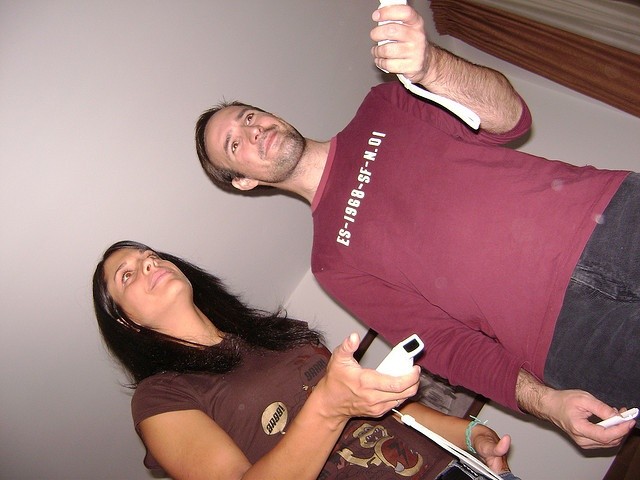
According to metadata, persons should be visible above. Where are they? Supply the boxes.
[195,6,639,453]
[93,241,512,480]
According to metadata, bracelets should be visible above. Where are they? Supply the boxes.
[465,414,489,456]
